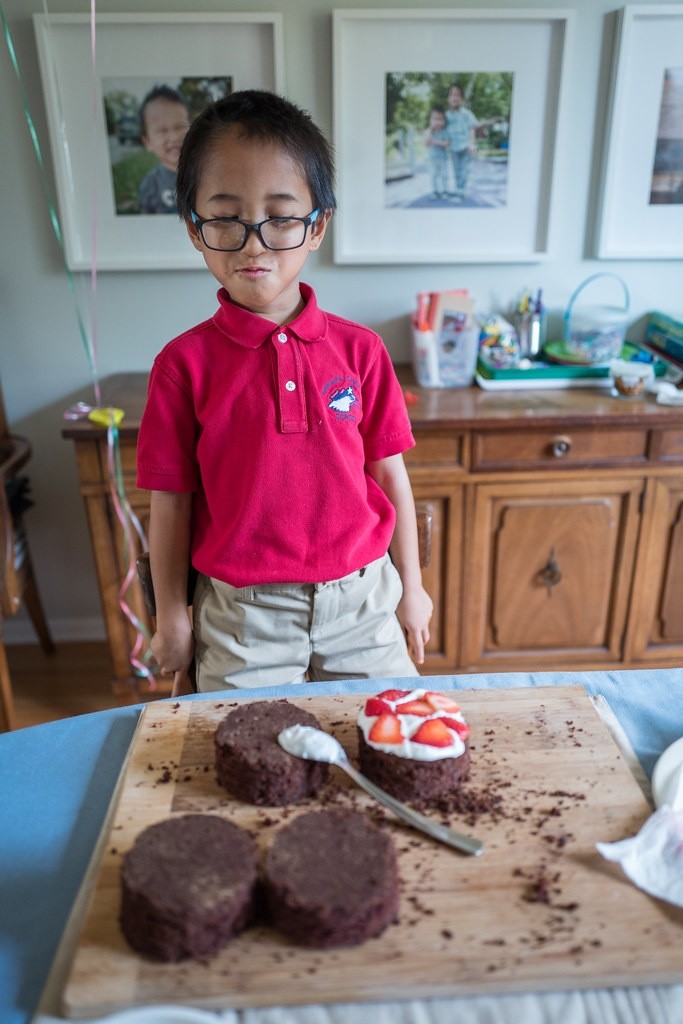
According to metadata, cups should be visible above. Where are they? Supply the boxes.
[517,314,543,358]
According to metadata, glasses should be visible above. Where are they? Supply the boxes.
[190,205,322,252]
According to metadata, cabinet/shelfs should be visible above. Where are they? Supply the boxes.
[456,342,682,673]
[61,369,463,706]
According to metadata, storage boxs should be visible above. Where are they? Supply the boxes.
[408,313,483,388]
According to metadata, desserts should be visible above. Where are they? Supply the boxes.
[356,689,470,807]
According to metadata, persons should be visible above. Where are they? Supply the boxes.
[422,104,450,201]
[134,81,198,213]
[129,86,436,696]
[420,81,482,199]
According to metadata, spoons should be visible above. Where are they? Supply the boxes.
[277,725,476,855]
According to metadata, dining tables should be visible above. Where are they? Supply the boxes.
[0,670,683,1024]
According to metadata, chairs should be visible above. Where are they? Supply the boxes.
[0,436,52,732]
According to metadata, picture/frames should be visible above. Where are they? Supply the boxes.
[331,5,577,267]
[29,12,284,273]
[594,5,683,262]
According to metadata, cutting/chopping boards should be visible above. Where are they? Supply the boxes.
[61,684,683,1017]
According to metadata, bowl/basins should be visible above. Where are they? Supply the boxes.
[612,359,655,399]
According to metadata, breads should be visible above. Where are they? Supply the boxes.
[262,807,398,948]
[115,814,259,962]
[214,698,325,806]
